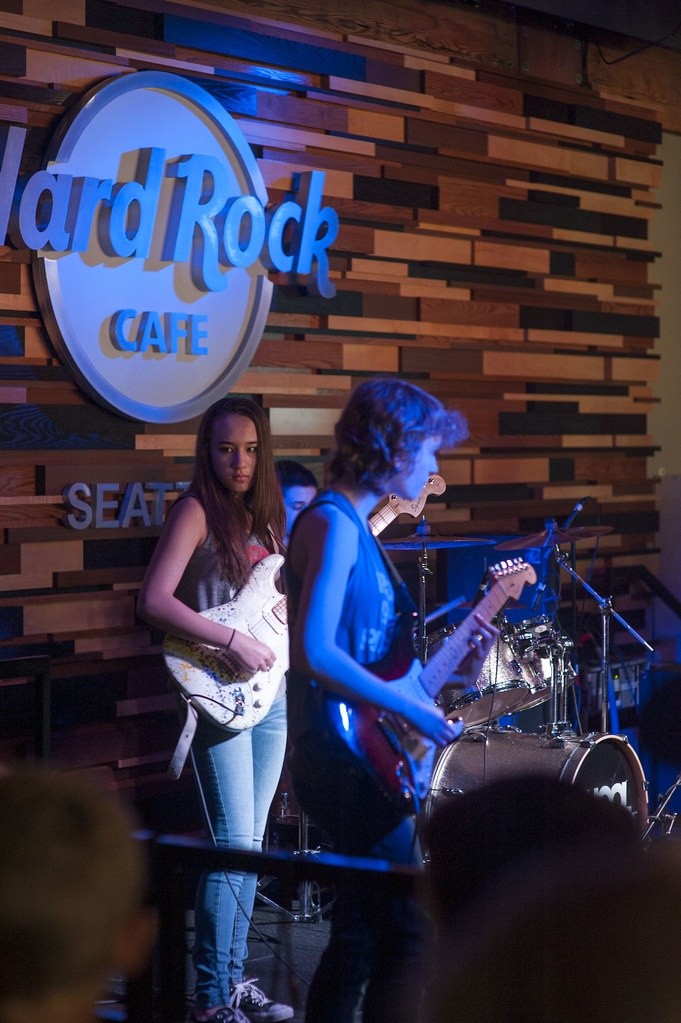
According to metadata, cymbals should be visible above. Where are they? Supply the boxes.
[372,531,495,549]
[493,525,615,552]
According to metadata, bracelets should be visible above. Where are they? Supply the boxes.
[226,629,235,650]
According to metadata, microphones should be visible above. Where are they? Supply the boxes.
[561,499,586,528]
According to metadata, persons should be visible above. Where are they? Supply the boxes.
[136,377,500,1023]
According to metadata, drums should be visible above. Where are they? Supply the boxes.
[417,727,650,887]
[409,617,580,730]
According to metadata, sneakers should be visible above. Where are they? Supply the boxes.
[189,979,296,1023]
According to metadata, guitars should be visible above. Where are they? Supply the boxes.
[315,556,538,817]
[161,473,448,733]
